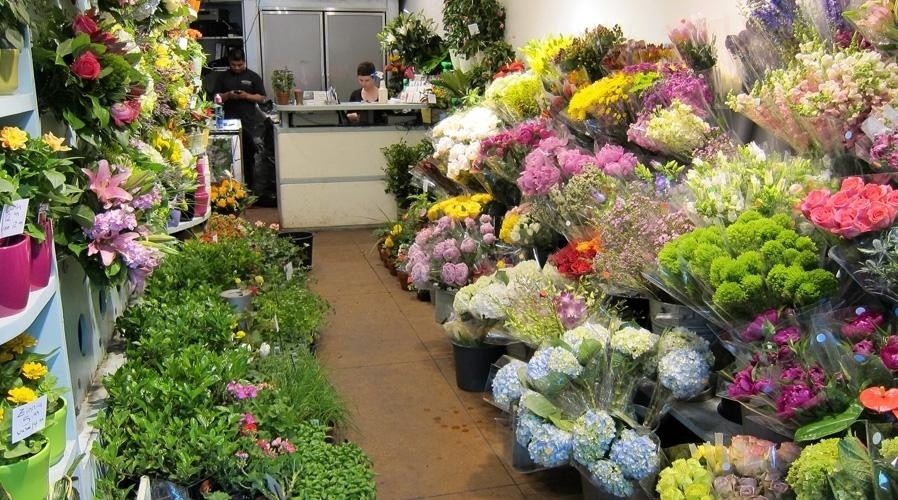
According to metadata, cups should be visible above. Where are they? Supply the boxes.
[294,88,304,106]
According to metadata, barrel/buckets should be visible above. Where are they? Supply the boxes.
[282,232,313,271]
[448,340,502,393]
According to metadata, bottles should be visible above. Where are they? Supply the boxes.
[377,80,388,104]
[214,92,225,130]
[402,78,409,92]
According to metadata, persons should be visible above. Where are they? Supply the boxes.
[345,61,379,122]
[213,48,266,188]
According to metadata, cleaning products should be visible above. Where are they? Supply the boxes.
[369,68,388,104]
